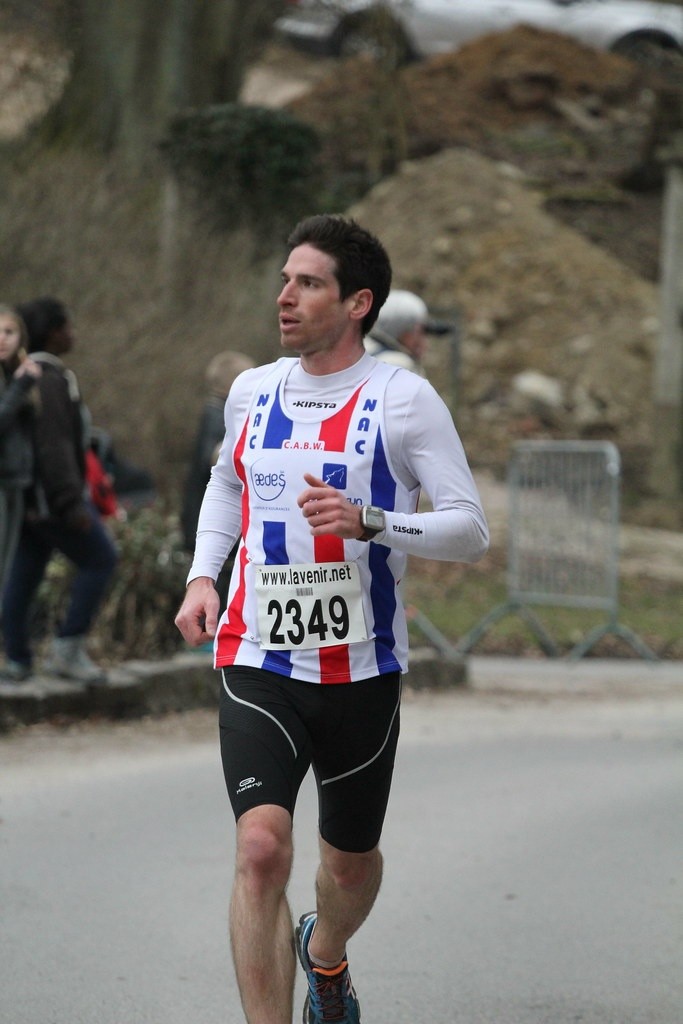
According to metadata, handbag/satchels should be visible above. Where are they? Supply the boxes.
[85,448,119,519]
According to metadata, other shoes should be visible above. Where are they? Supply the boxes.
[6,655,46,680]
[50,636,108,686]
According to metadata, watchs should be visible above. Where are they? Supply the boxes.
[356,505,385,542]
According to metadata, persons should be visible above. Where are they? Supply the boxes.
[86,438,119,516]
[181,350,255,561]
[1,296,121,683]
[363,290,428,381]
[173,214,490,1024]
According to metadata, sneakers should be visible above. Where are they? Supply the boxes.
[295,911,361,1024]
